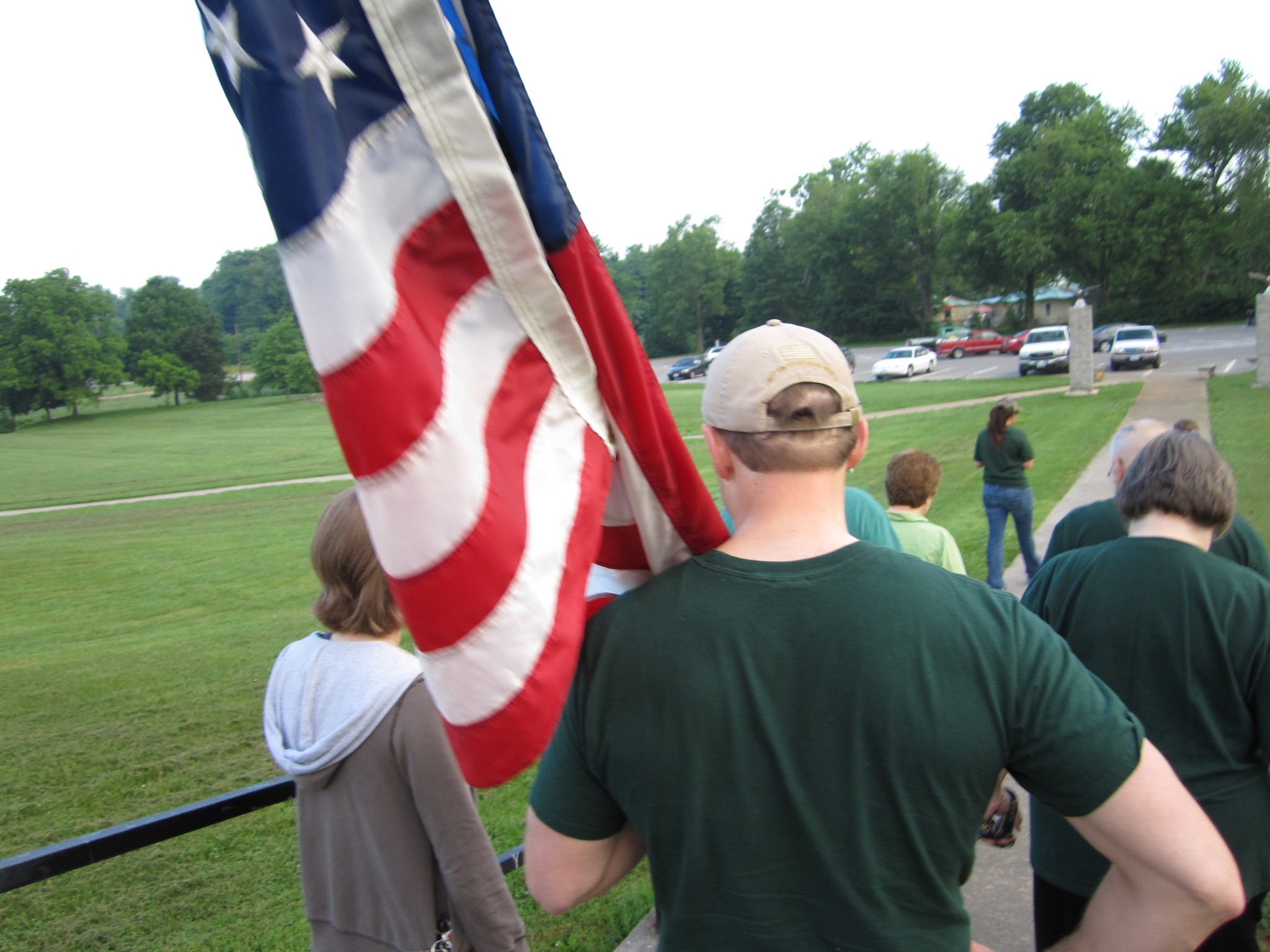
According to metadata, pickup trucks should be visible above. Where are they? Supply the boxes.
[935,330,1012,358]
[906,324,971,351]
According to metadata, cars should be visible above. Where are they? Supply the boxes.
[702,346,724,365]
[667,356,709,380]
[1018,324,1072,375]
[872,346,939,381]
[999,328,1032,355]
[838,346,856,375]
[1092,320,1169,352]
[1108,326,1163,370]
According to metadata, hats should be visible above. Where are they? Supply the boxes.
[701,318,863,433]
[996,396,1023,411]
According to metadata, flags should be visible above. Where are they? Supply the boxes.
[195,0,732,792]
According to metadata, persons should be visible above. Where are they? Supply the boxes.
[980,417,1270,952]
[524,319,1245,952]
[264,486,533,952]
[719,486,904,555]
[885,450,967,576]
[972,397,1042,591]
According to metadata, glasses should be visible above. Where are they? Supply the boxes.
[1106,461,1114,483]
